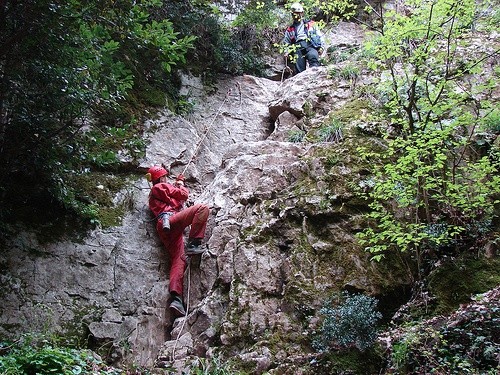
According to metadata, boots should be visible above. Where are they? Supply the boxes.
[187,237,207,255]
[169,291,186,317]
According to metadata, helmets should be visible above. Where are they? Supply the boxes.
[148,166,169,182]
[291,2,305,13]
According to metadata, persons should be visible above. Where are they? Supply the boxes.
[146,166,210,316]
[282,2,324,76]
